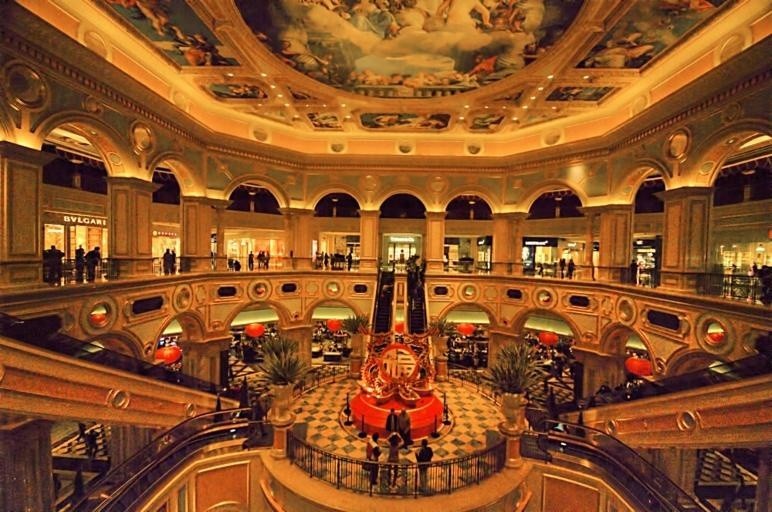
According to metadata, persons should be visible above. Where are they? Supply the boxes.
[732,262,772,304]
[48,245,102,283]
[316,251,352,270]
[53,473,61,499]
[248,251,270,271]
[415,439,433,488]
[74,469,84,498]
[76,423,99,458]
[367,433,382,484]
[384,432,405,488]
[233,260,241,271]
[560,258,575,279]
[245,395,265,437]
[386,408,410,449]
[631,260,639,285]
[163,249,176,275]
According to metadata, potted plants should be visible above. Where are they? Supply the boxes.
[246,333,308,424]
[482,341,549,427]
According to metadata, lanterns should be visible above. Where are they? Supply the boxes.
[326,319,340,330]
[459,325,474,334]
[625,357,651,376]
[91,315,105,322]
[155,346,183,365]
[245,323,264,336]
[539,331,558,345]
